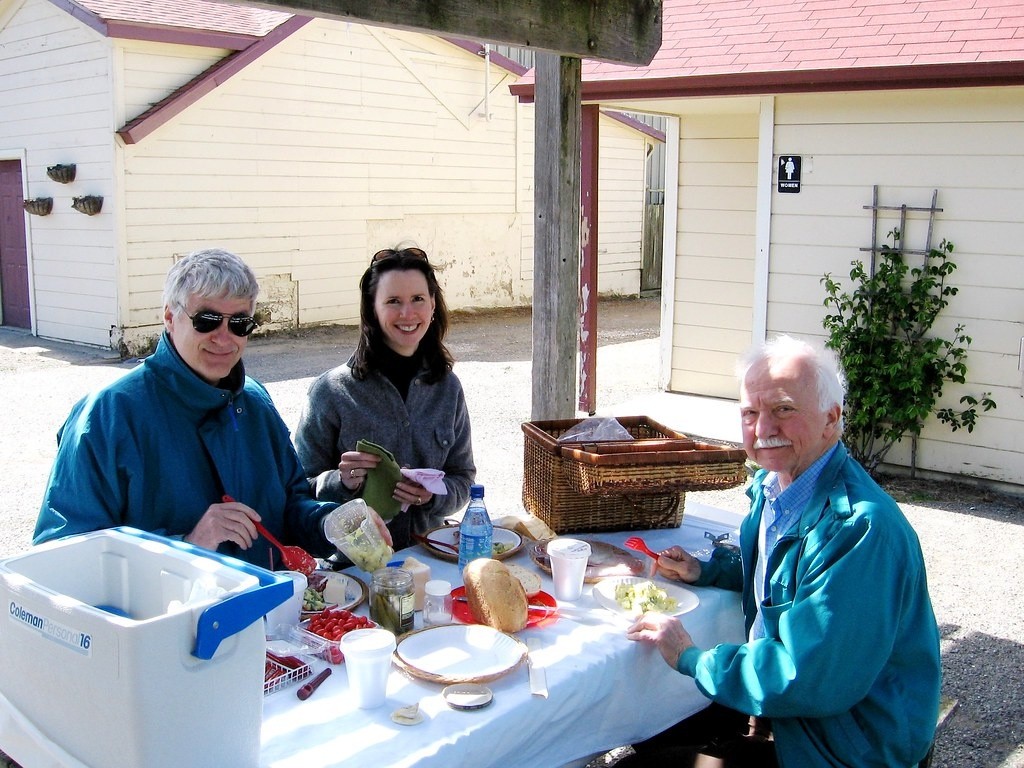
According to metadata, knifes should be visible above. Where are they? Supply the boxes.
[455,596,560,611]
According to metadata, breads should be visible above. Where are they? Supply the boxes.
[400,556,431,614]
[463,557,529,633]
[501,563,543,596]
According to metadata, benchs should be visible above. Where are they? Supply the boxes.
[919,696,960,768]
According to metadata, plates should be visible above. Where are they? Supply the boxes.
[445,584,557,629]
[395,623,523,676]
[425,524,531,556]
[592,576,699,619]
[292,571,363,613]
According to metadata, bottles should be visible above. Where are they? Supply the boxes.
[369,568,416,634]
[458,484,493,577]
[422,580,453,625]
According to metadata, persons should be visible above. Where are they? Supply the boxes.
[32,245,393,572]
[292,237,477,569]
[628,333,942,768]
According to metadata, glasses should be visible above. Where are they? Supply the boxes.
[370,248,429,280]
[177,301,257,337]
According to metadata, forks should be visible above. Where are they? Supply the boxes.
[624,537,660,561]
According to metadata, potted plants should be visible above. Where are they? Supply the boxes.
[47,163,75,184]
[22,196,53,216]
[71,193,104,216]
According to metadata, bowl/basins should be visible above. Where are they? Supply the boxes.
[290,608,381,664]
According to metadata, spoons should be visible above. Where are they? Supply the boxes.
[222,494,317,578]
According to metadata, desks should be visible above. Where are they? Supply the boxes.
[0,512,743,768]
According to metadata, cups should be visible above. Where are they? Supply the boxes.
[546,538,593,602]
[339,628,397,710]
[264,569,309,639]
[324,497,392,574]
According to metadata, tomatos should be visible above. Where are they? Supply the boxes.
[305,608,377,663]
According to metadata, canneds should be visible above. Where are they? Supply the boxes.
[371,568,416,635]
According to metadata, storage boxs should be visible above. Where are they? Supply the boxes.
[0,533,292,768]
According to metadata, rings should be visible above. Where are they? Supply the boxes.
[351,469,357,478]
[414,497,422,505]
